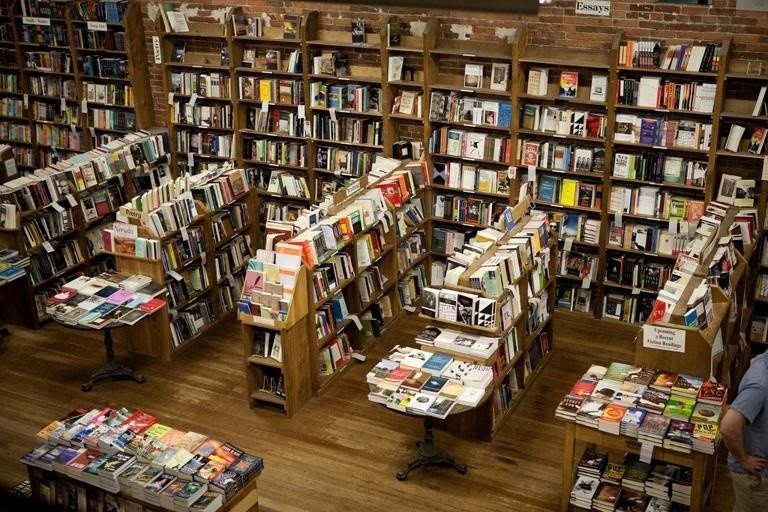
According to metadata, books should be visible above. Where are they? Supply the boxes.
[1,129,171,324]
[365,184,554,429]
[156,3,382,224]
[19,405,264,511]
[554,361,729,511]
[383,21,717,325]
[46,159,254,349]
[234,157,427,397]
[648,87,768,368]
[0,1,135,179]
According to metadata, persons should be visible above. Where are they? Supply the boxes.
[720,352,768,511]
